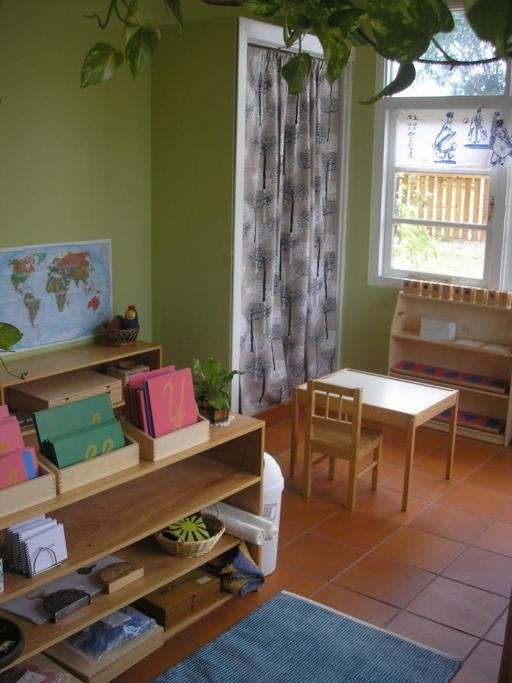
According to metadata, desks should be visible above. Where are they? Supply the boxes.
[291,368,460,512]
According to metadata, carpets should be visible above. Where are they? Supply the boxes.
[152,588,464,683]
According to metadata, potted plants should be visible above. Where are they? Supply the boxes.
[191,356,247,424]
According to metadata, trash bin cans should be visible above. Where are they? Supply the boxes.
[260,451,284,577]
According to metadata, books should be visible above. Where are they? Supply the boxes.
[1,515,68,576]
[418,315,457,342]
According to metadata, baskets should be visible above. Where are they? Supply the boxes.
[99,321,142,347]
[155,502,227,559]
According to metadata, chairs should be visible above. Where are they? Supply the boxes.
[301,379,383,511]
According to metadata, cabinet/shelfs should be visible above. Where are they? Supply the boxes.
[0,340,266,682]
[383,279,512,448]
